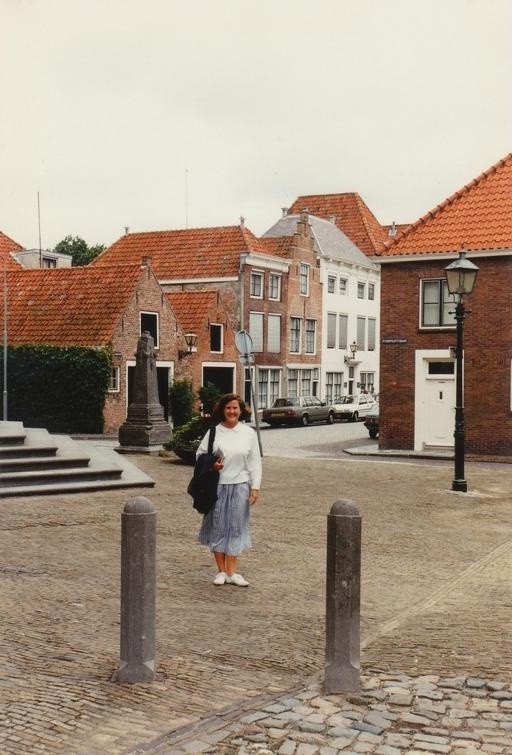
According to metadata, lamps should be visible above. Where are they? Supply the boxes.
[178,329,197,358]
[342,341,358,363]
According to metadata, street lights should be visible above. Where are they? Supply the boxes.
[441,248,480,493]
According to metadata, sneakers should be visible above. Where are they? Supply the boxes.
[226,574,249,586]
[213,572,226,585]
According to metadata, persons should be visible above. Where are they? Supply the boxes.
[195,394,262,587]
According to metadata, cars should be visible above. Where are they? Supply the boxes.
[261,395,336,426]
[364,404,379,439]
[329,393,379,422]
